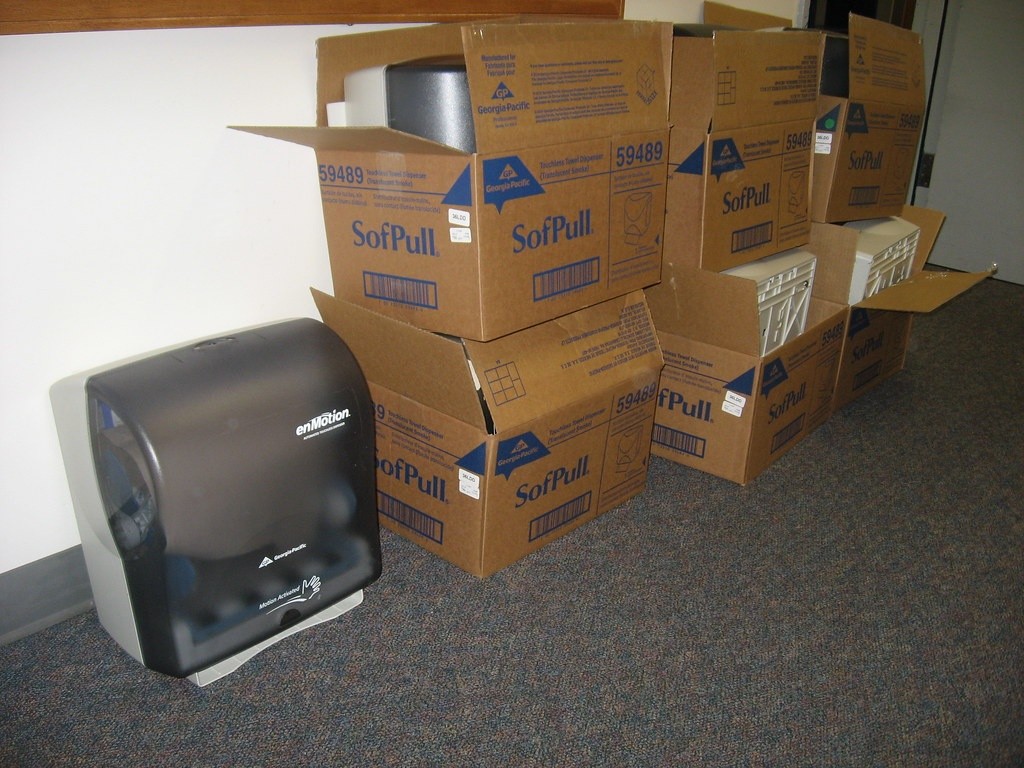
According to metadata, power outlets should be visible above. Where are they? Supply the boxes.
[917,152,934,187]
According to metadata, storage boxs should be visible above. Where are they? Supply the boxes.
[702,0,927,226]
[840,213,922,304]
[661,21,828,276]
[307,287,667,584]
[833,204,994,411]
[721,247,817,356]
[217,17,676,343]
[644,218,860,489]
